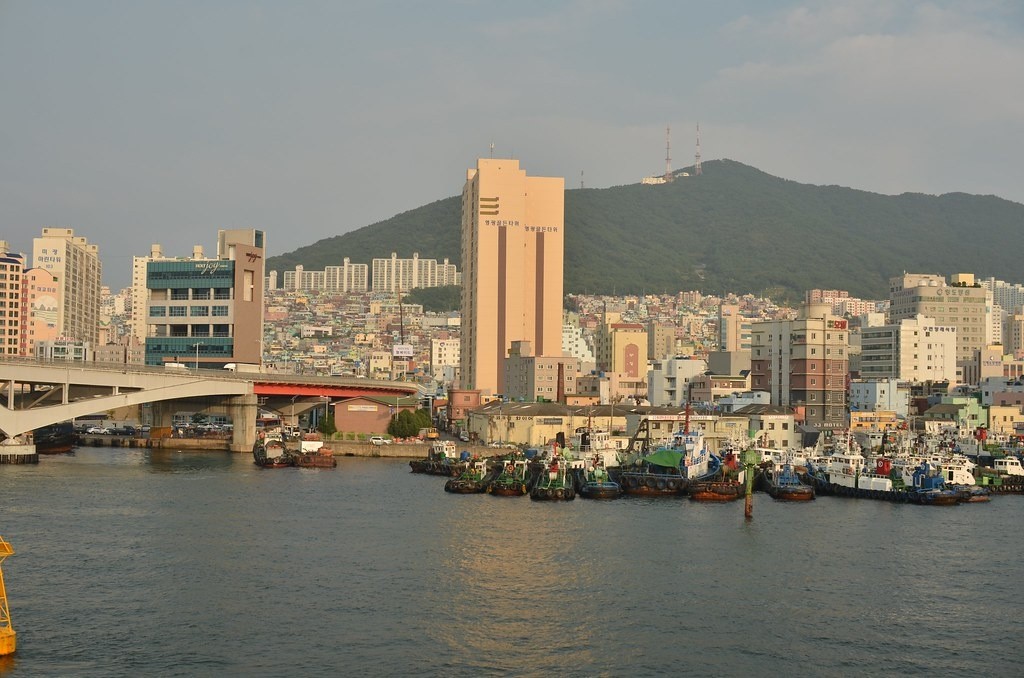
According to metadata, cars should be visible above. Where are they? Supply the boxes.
[75,423,96,434]
[86,427,105,435]
[137,424,151,433]
[427,428,440,440]
[460,431,470,441]
[100,428,115,435]
[491,441,516,449]
[370,436,393,446]
[177,422,233,431]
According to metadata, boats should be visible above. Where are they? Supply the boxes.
[409,440,463,477]
[442,405,1023,502]
[252,424,338,471]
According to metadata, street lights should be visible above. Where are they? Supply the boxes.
[396,396,410,422]
[193,342,204,368]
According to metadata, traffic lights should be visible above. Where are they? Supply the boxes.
[174,356,177,361]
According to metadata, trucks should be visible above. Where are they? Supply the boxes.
[113,426,135,436]
[224,363,260,374]
[165,363,191,374]
